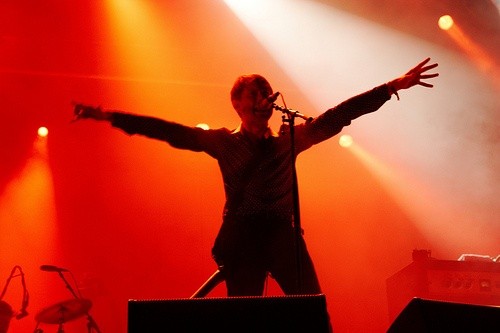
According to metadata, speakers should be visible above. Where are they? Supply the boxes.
[126,294,333,333]
[386,297,500,333]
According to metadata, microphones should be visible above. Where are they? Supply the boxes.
[40,265,67,272]
[16,312,27,320]
[260,92,279,110]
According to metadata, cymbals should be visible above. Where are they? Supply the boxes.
[34,296,93,326]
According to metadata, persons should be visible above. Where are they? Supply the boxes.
[71,58,439,297]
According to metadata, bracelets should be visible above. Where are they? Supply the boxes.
[386,80,399,100]
